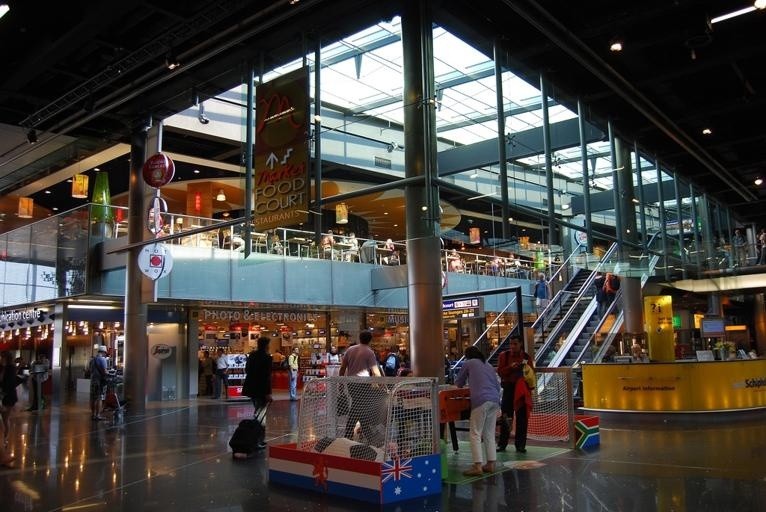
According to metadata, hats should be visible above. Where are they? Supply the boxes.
[98,345,107,352]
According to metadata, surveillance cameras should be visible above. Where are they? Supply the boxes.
[199,113,209,124]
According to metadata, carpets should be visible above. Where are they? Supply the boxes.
[438,439,570,487]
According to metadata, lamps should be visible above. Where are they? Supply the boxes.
[0,308,120,341]
[22,48,179,146]
[216,190,227,203]
[195,318,326,339]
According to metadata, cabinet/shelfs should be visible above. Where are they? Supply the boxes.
[281,328,339,396]
[224,353,250,401]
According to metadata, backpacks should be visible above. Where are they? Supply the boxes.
[281,355,296,369]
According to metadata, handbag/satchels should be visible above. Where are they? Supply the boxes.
[336,392,349,416]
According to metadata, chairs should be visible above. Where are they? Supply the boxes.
[111,220,536,278]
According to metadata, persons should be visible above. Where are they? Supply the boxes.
[605,345,619,362]
[272,348,288,370]
[533,273,552,321]
[240,336,273,448]
[286,401,299,445]
[464,475,498,512]
[732,229,747,268]
[237,455,272,512]
[453,344,501,477]
[326,330,452,450]
[232,218,529,277]
[0,345,114,465]
[630,342,649,362]
[287,347,299,401]
[197,348,233,400]
[496,333,534,455]
[593,254,624,324]
[501,454,534,512]
[754,227,766,266]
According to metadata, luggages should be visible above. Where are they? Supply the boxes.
[229,398,273,458]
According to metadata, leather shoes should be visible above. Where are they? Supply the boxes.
[255,443,266,450]
[96,416,106,420]
[496,446,505,452]
[483,464,495,472]
[516,447,526,453]
[464,468,483,475]
[91,415,96,420]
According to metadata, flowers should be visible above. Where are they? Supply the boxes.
[712,339,735,352]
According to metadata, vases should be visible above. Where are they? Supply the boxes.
[720,350,730,361]
